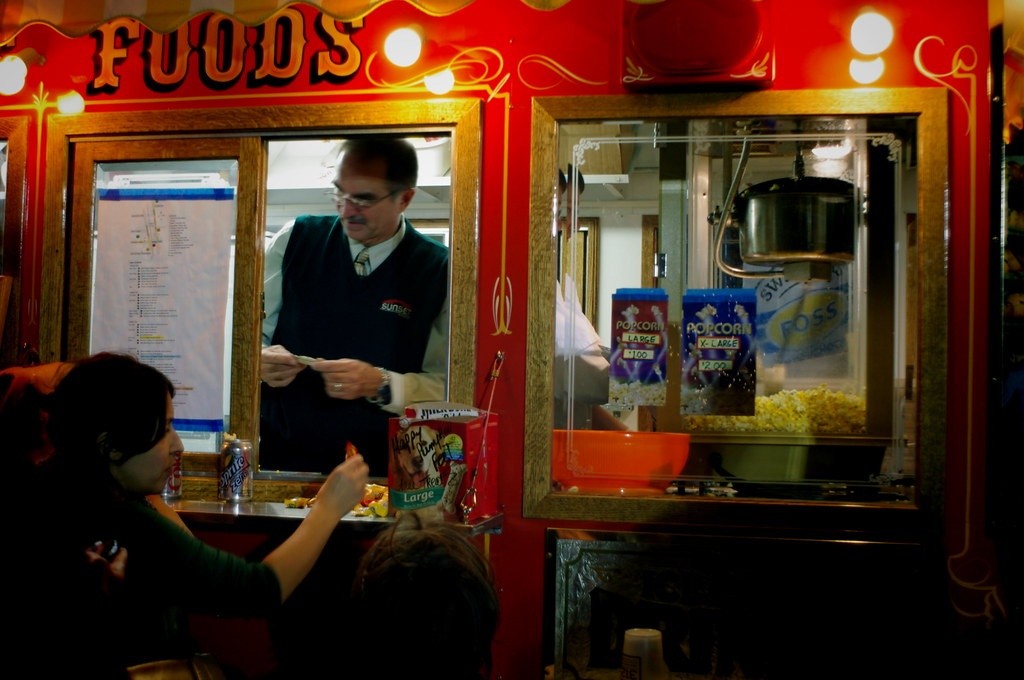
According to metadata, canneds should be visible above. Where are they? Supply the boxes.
[160,450,182,500]
[218,438,255,504]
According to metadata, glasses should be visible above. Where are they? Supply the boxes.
[324,188,402,205]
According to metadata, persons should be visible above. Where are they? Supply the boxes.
[554,163,610,428]
[259,140,449,477]
[0,354,370,680]
[353,508,498,680]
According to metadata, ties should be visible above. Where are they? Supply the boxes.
[353,248,368,276]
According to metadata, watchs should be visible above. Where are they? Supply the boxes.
[367,366,392,406]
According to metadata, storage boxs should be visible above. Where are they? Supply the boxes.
[388,402,497,524]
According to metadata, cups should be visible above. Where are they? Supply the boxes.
[619,628,664,680]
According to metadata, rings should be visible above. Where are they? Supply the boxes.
[334,383,343,392]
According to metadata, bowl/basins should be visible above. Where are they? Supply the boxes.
[553,428,693,498]
